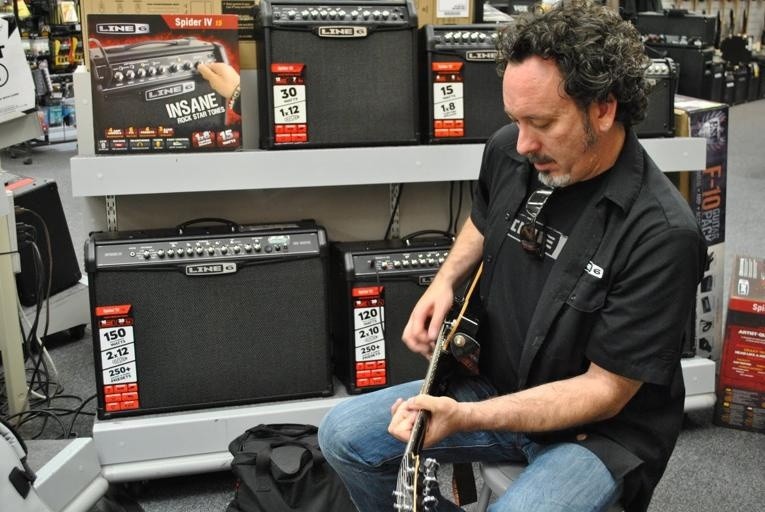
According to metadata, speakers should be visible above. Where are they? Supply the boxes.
[85,217,334,418]
[330,230,481,395]
[4,171,81,306]
[633,58,676,138]
[256,0,423,149]
[418,23,513,142]
[637,12,765,108]
[89,36,242,153]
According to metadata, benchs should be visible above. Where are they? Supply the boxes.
[475,462,528,512]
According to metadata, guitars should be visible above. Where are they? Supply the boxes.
[391,260,483,512]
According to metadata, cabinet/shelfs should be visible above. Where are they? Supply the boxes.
[67,137,719,484]
[0,113,110,512]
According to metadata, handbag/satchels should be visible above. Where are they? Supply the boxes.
[224,423,357,512]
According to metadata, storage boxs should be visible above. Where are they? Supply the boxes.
[78,0,619,75]
[671,92,765,434]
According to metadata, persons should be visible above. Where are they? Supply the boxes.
[318,3,707,512]
[196,60,242,117]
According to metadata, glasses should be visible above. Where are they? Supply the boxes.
[520,185,557,260]
